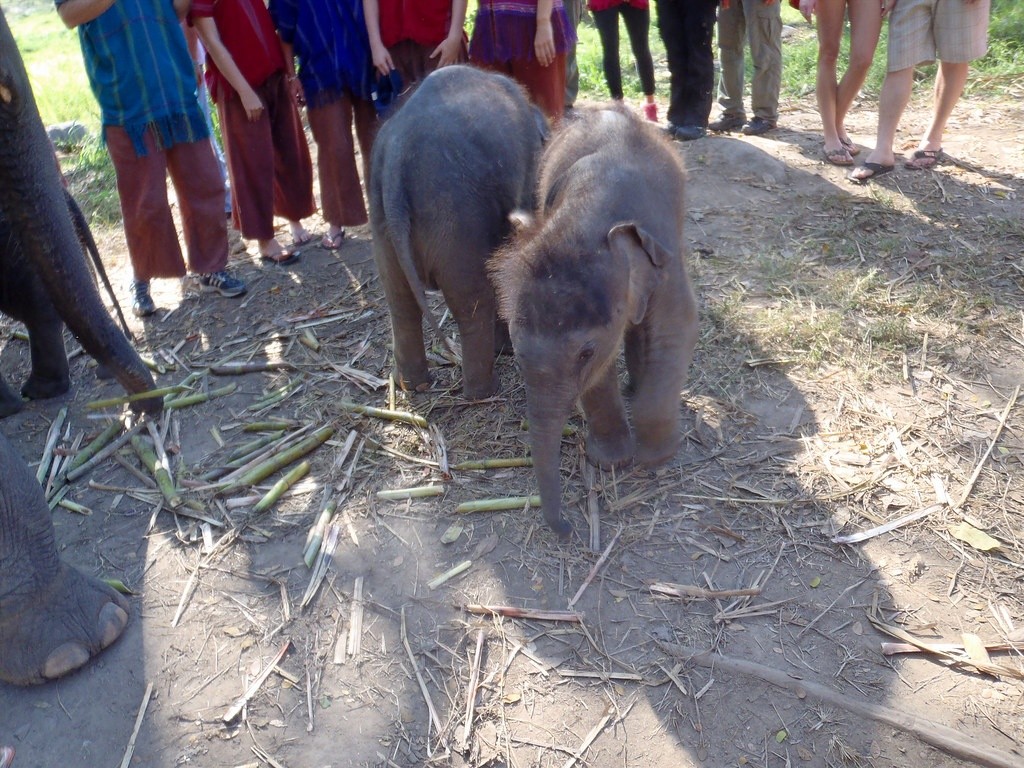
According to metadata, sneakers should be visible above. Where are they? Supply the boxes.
[741,117,777,135]
[668,123,707,139]
[709,114,747,131]
[199,271,247,298]
[130,278,156,315]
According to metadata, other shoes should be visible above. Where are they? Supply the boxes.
[642,102,658,122]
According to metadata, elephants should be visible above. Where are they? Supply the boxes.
[507,106,702,531]
[0,11,164,683]
[368,65,550,399]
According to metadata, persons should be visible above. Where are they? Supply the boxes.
[56,0,783,314]
[847,0,992,182]
[790,0,897,166]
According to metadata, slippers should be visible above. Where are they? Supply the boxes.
[848,160,894,184]
[291,229,312,244]
[839,137,860,156]
[259,247,301,266]
[903,147,943,169]
[322,228,346,250]
[822,145,855,166]
